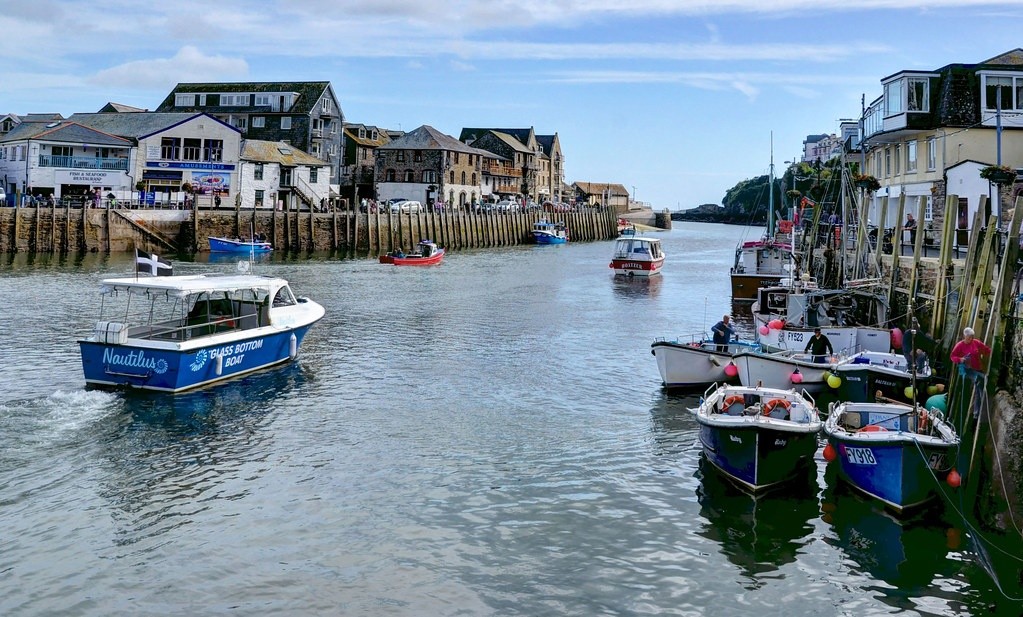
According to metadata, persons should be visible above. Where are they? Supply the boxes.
[430,200,435,215]
[25,189,101,209]
[215,194,220,211]
[385,199,388,214]
[950,327,990,419]
[235,192,242,212]
[185,193,188,210]
[804,327,833,363]
[711,314,734,352]
[902,214,917,254]
[902,324,919,374]
[360,198,377,214]
[191,193,196,209]
[393,248,406,258]
[320,197,327,213]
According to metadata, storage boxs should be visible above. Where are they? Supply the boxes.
[95,321,131,345]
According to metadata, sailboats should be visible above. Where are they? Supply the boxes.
[726,131,893,362]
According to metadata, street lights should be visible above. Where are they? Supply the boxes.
[784,157,796,207]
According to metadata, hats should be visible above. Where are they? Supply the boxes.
[814,327,821,333]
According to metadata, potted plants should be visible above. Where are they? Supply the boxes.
[855,173,882,193]
[978,164,1017,187]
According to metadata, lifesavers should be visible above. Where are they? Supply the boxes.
[917,409,928,434]
[764,398,791,418]
[780,220,793,233]
[722,396,744,413]
[856,425,888,432]
[215,317,233,327]
[689,342,700,347]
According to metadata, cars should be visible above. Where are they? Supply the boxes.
[388,200,421,216]
[365,198,408,214]
[475,198,597,215]
[0,187,6,207]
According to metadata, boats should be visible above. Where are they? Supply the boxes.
[207,232,273,254]
[530,215,570,244]
[609,233,665,276]
[379,239,446,265]
[729,346,843,402]
[824,388,961,525]
[74,221,327,395]
[685,381,823,500]
[619,224,638,238]
[831,348,932,407]
[651,297,765,390]
